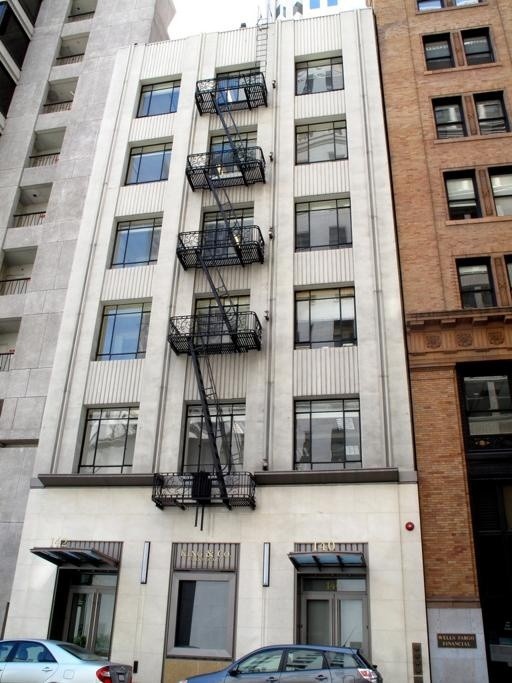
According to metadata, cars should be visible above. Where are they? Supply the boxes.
[178,643,384,682]
[0,638,134,682]
